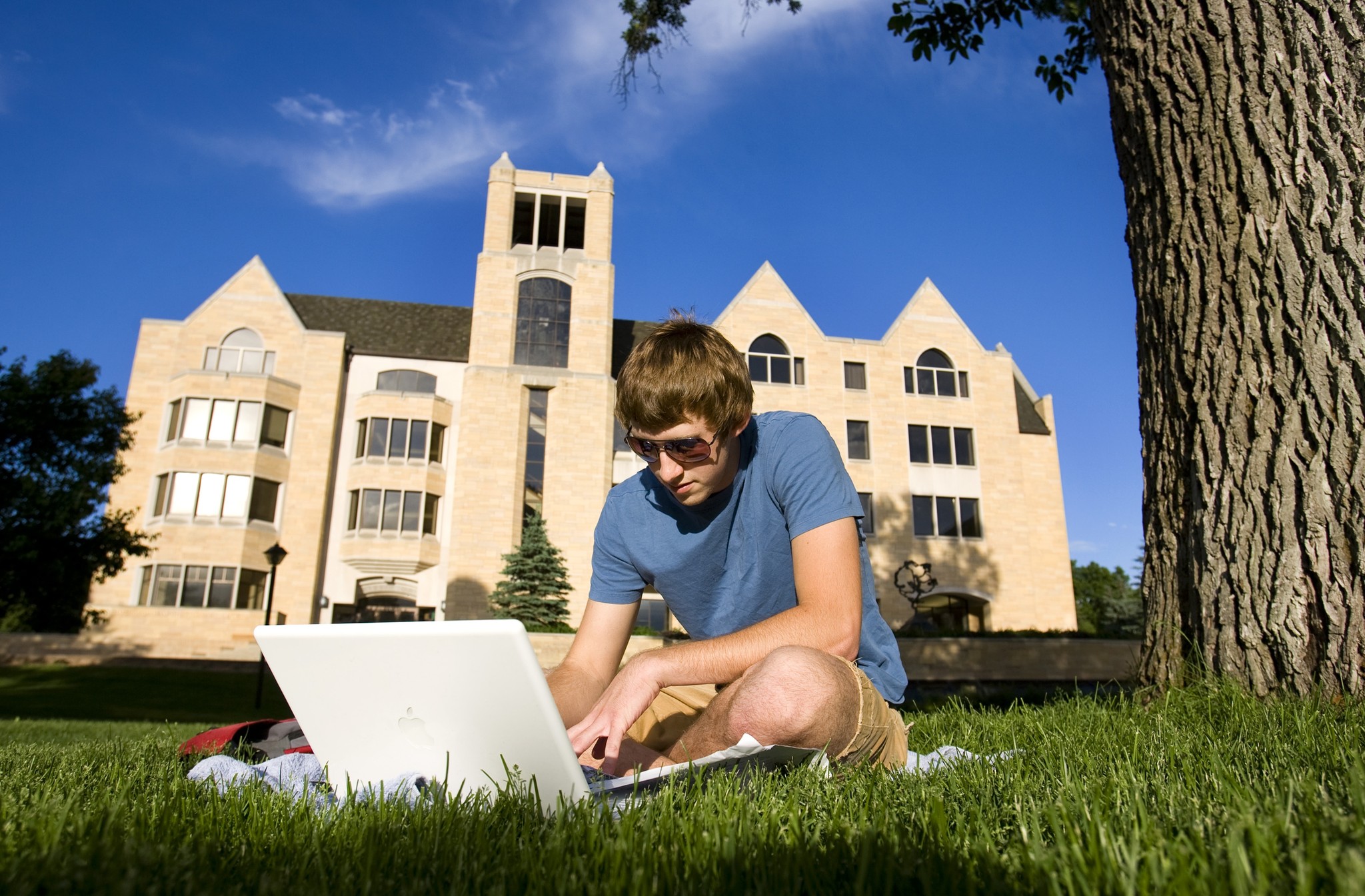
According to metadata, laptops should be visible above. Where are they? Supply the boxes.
[253,617,661,830]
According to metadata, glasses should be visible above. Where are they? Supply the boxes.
[624,425,724,464]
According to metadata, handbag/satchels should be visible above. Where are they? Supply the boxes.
[176,717,314,781]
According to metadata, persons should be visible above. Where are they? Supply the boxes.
[543,321,909,777]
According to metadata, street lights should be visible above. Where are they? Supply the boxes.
[261,541,288,706]
